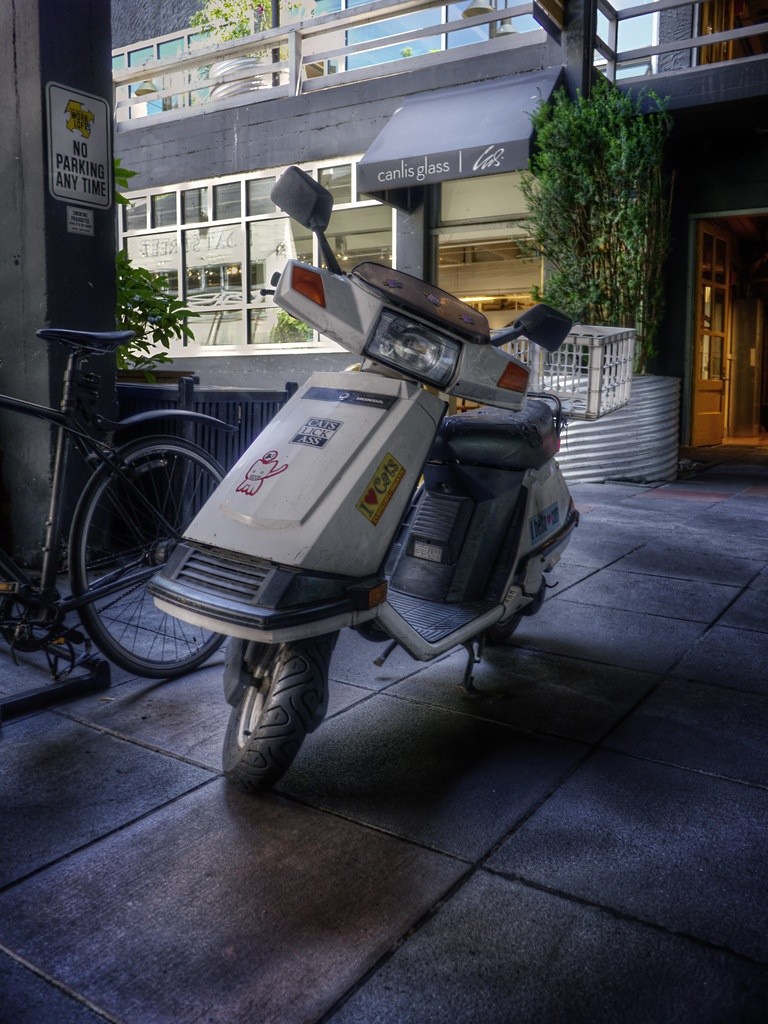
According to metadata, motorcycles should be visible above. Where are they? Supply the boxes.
[143,166,584,792]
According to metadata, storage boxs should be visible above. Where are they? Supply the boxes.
[529,326,636,419]
[487,329,527,366]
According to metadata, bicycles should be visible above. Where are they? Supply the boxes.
[0,328,241,705]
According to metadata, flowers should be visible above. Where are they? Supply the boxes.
[189,0,301,42]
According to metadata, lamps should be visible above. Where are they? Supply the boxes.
[462,0,494,19]
[496,0,516,36]
[134,55,157,96]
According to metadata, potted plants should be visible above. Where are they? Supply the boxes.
[512,70,681,486]
[114,156,196,386]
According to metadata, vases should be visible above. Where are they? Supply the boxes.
[208,56,265,100]
